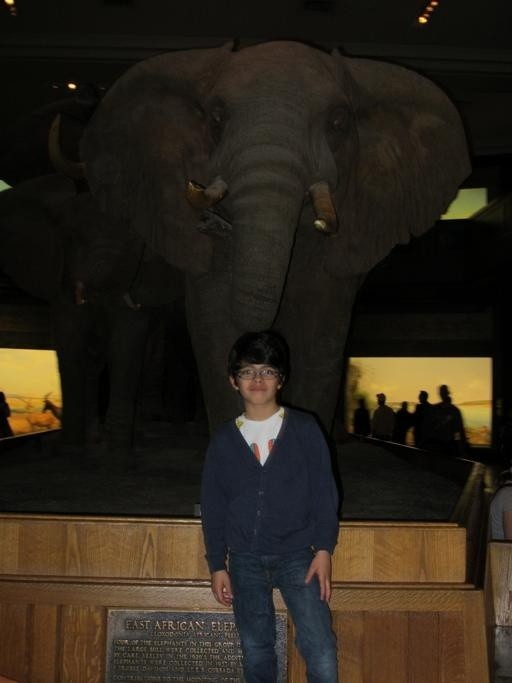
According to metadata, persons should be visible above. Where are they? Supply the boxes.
[0,392,15,438]
[200,331,340,683]
[352,385,471,447]
[488,486,512,539]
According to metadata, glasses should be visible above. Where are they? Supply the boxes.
[233,367,279,380]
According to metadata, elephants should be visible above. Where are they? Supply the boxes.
[1,39,472,473]
